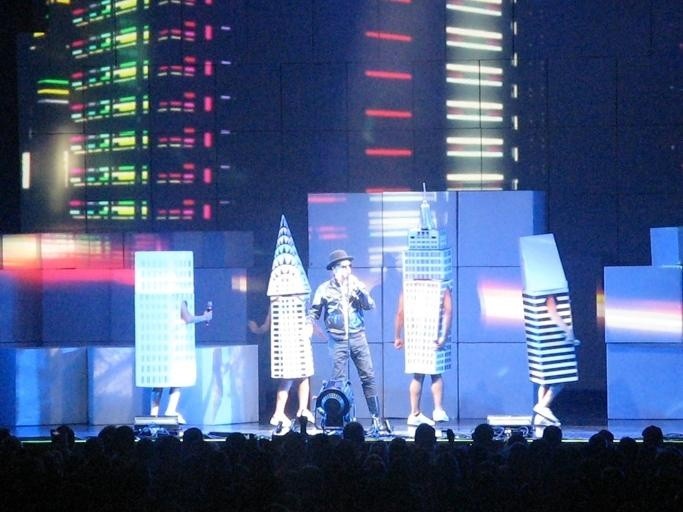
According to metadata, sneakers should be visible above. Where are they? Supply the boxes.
[270,415,291,428]
[294,410,316,424]
[433,409,449,422]
[165,412,187,424]
[407,414,436,427]
[533,404,560,426]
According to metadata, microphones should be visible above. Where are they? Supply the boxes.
[566,336,581,348]
[435,338,448,354]
[206,301,214,322]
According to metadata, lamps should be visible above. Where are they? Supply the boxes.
[316,381,355,430]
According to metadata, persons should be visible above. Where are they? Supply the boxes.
[393,283,453,426]
[247,305,315,427]
[149,302,213,425]
[532,295,574,439]
[0,423,683,512]
[308,250,387,431]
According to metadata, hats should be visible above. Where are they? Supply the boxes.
[326,248,354,269]
